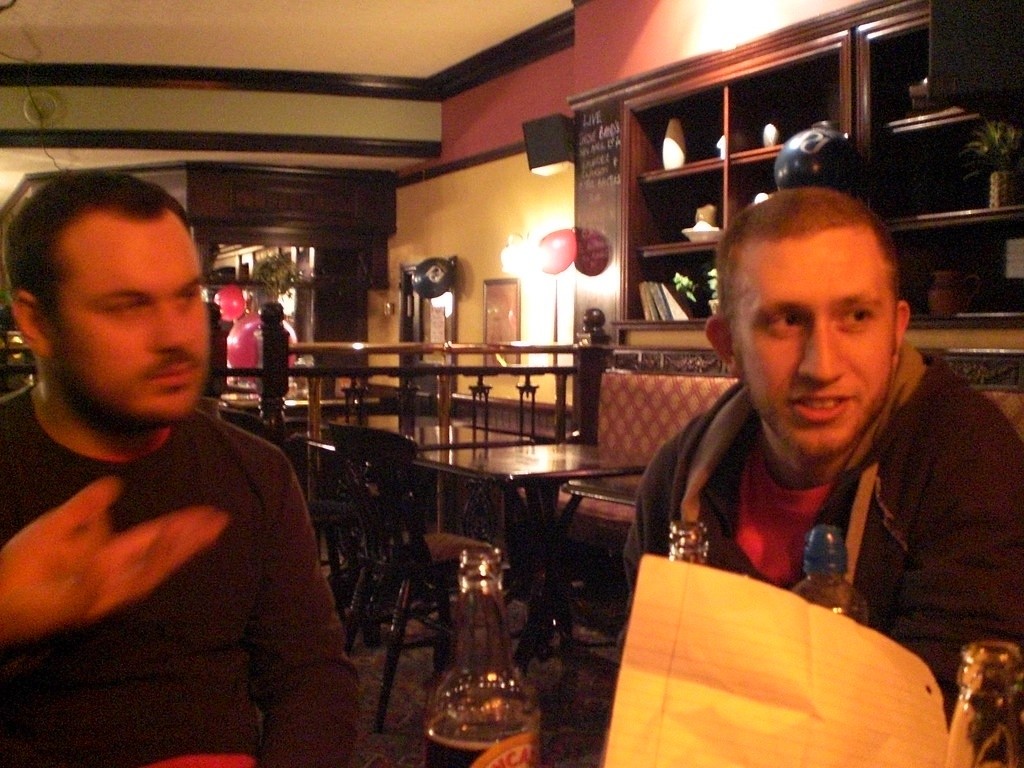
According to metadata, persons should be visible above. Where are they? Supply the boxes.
[0,476,231,642]
[617,189,1024,732]
[0,171,358,768]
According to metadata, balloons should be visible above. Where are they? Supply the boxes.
[535,229,576,273]
[226,314,299,380]
[775,127,864,196]
[213,285,246,323]
[410,257,454,299]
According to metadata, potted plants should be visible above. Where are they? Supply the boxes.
[962,119,1022,210]
[672,258,719,318]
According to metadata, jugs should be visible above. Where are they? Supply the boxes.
[927,271,981,316]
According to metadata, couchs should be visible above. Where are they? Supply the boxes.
[558,344,1024,552]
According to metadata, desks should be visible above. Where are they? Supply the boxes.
[382,427,533,446]
[226,397,382,413]
[338,413,468,432]
[412,442,652,639]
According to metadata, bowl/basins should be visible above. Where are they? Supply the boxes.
[682,227,720,242]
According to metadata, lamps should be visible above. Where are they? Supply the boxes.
[520,109,573,176]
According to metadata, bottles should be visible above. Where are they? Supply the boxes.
[668,521,708,563]
[944,639,1023,768]
[424,546,539,768]
[791,525,868,627]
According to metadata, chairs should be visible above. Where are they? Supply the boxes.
[222,411,511,732]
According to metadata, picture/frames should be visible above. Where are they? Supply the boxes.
[413,283,458,367]
[483,278,521,365]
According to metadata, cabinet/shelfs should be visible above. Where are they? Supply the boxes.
[191,229,370,401]
[625,1,1023,331]
